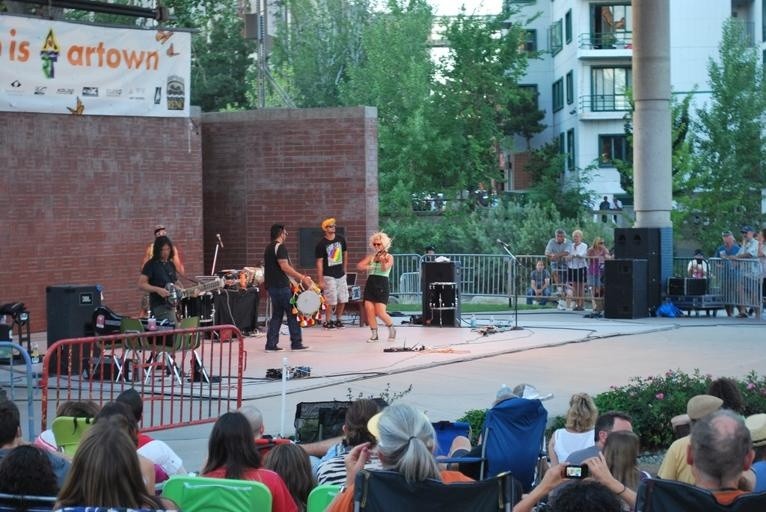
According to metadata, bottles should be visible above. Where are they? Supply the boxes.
[281,357,290,382]
[471,314,476,327]
[489,315,494,324]
[31,341,40,362]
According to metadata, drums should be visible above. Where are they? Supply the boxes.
[295,290,322,314]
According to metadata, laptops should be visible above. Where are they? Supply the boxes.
[346,272,357,286]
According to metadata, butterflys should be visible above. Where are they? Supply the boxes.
[167,43,180,57]
[156,29,173,45]
[66,96,85,116]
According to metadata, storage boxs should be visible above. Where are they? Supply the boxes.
[87,356,134,381]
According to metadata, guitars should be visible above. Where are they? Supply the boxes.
[165,280,222,304]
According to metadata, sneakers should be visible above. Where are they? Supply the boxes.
[557,300,567,310]
[291,345,310,351]
[265,345,283,352]
[323,320,345,330]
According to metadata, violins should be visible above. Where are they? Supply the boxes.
[374,250,391,264]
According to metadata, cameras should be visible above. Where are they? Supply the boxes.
[565,463,588,479]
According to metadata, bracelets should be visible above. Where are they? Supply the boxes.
[301,275,306,283]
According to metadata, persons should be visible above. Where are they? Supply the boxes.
[315,217,349,329]
[447,384,766,512]
[612,197,622,223]
[0,387,187,511]
[419,245,437,291]
[599,195,610,222]
[357,229,397,342]
[689,227,765,317]
[140,225,184,277]
[526,228,615,311]
[199,397,478,511]
[138,235,200,331]
[264,223,314,352]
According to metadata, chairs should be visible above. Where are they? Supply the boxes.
[635,478,765,511]
[306,485,346,511]
[445,397,550,509]
[161,476,274,511]
[357,467,515,511]
[60,507,157,512]
[281,402,352,444]
[316,399,389,442]
[51,415,99,460]
[90,306,135,383]
[115,318,177,384]
[1,492,58,511]
[425,422,471,459]
[145,314,210,387]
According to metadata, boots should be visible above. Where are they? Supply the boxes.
[387,323,396,341]
[367,329,379,344]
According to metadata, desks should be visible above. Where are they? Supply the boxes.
[333,298,368,327]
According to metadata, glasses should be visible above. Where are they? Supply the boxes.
[326,224,336,228]
[373,243,383,246]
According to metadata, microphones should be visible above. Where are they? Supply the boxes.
[496,239,511,250]
[216,233,224,247]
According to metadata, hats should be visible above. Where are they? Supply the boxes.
[741,226,753,232]
[687,394,723,419]
[745,413,765,446]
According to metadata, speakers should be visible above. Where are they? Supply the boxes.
[604,258,648,319]
[245,14,260,39]
[46,284,101,375]
[614,228,661,312]
[421,262,462,328]
[87,357,142,382]
